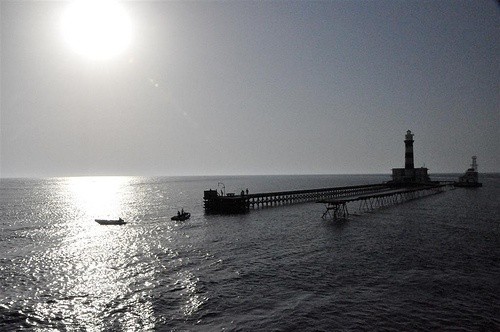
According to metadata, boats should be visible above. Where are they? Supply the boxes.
[171,212,190,221]
[94,220,127,225]
[456,156,483,186]
